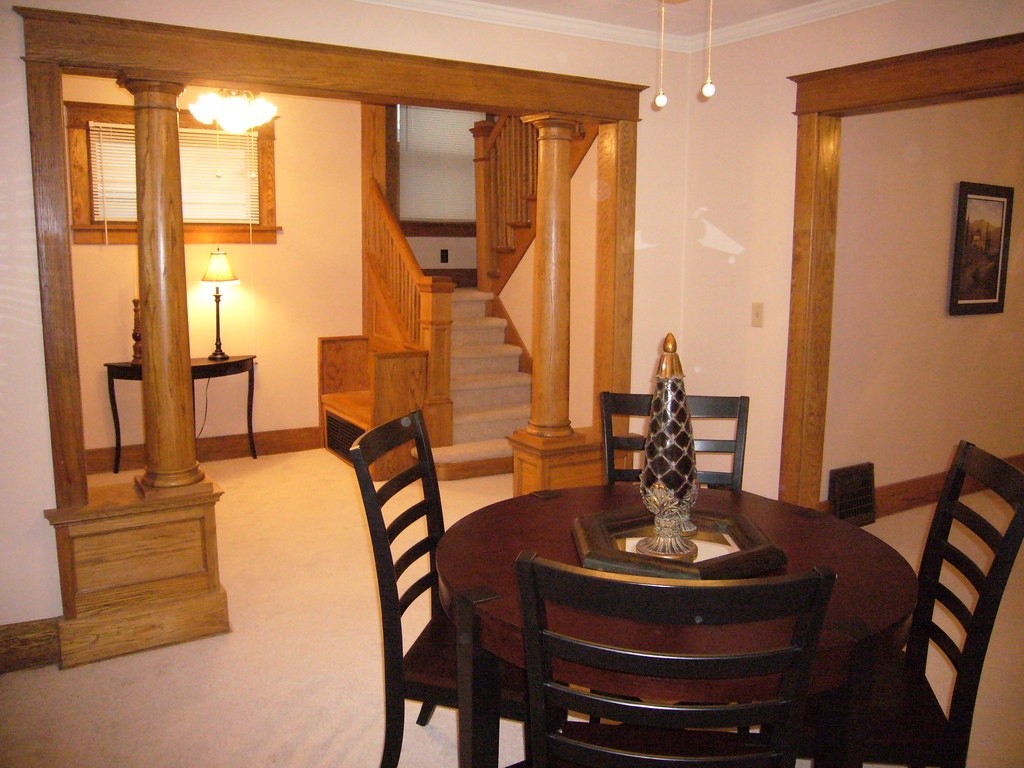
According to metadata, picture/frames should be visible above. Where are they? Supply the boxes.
[948,181,1014,316]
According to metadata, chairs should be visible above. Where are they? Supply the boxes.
[348,407,568,768]
[589,390,751,735]
[514,548,838,768]
[760,440,1024,768]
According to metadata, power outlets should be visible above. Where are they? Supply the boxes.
[440,250,448,263]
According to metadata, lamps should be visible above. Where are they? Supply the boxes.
[187,86,278,180]
[200,247,240,362]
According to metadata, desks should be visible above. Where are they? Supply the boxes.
[103,354,258,474]
[441,484,919,768]
